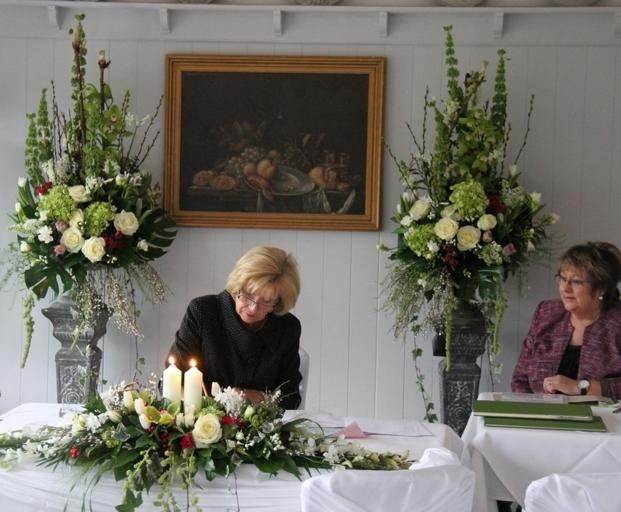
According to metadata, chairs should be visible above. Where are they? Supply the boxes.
[521,471,604,512]
[293,462,479,512]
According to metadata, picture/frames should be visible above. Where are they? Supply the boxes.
[155,47,388,234]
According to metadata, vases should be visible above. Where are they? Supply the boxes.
[427,277,488,437]
[36,284,116,405]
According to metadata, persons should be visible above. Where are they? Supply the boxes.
[511,239,621,397]
[165,246,305,412]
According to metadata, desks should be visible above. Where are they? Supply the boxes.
[0,398,468,512]
[454,386,621,512]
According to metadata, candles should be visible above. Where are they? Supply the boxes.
[181,357,205,417]
[159,354,183,408]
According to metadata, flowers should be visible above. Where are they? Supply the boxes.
[0,379,414,512]
[1,16,179,372]
[373,24,571,425]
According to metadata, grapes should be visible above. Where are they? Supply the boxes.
[227,144,312,178]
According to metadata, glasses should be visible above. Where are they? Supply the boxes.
[237,288,282,313]
[552,268,598,289]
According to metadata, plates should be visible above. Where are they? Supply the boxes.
[243,166,316,197]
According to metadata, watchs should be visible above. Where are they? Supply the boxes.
[577,376,593,396]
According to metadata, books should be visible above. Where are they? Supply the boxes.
[483,416,608,432]
[471,398,595,422]
